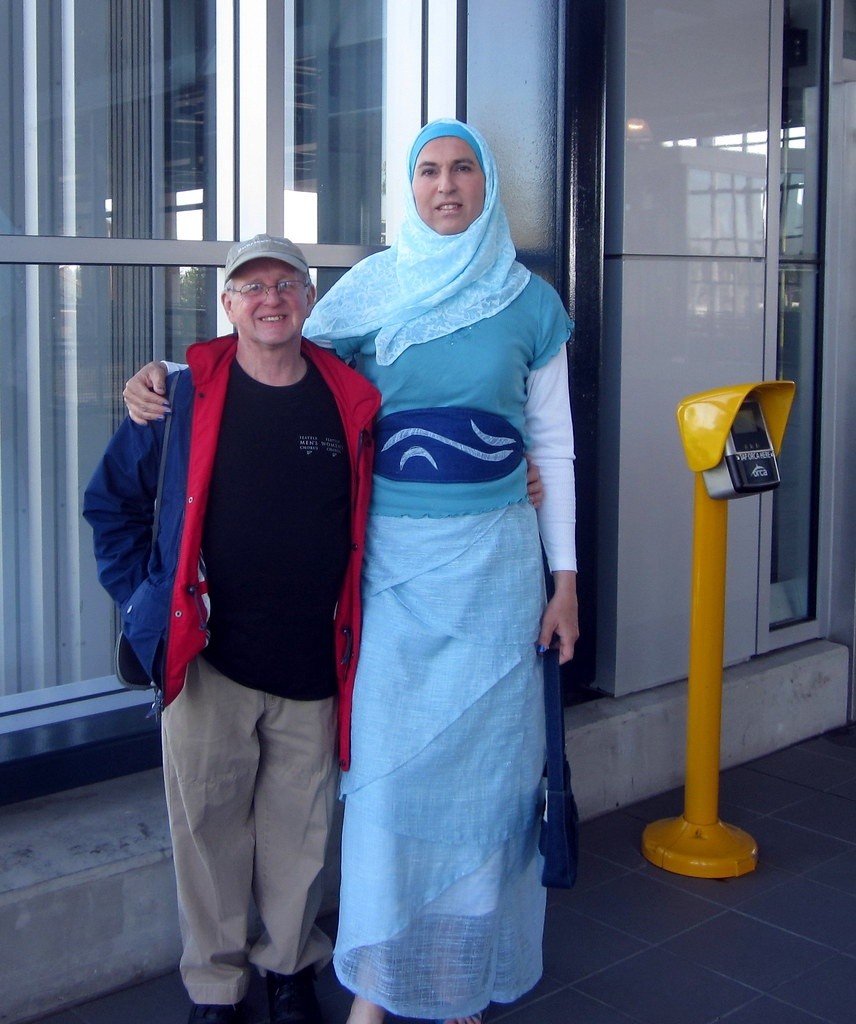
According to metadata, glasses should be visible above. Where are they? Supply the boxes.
[228,282,308,303]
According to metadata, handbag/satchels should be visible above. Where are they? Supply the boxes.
[119,638,152,692]
[539,761,584,888]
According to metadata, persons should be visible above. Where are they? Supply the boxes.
[83,233,382,1023]
[124,120,583,1023]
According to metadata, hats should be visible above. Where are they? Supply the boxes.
[226,234,310,281]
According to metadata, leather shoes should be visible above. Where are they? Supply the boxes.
[267,965,319,1024]
[187,1003,248,1023]
